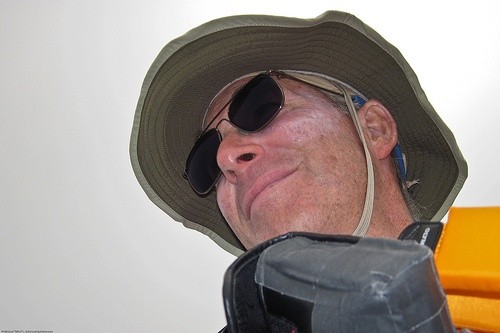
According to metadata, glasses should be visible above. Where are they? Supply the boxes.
[182,69,355,198]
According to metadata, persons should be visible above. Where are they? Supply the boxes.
[129,10,469,333]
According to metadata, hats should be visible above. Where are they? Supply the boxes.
[128,8,469,256]
